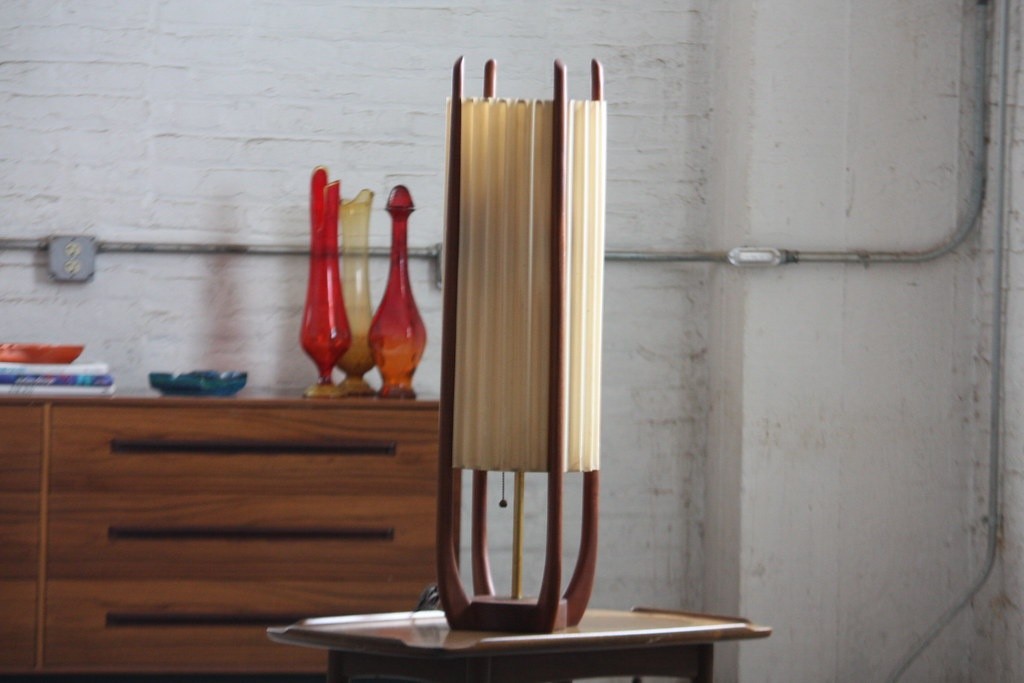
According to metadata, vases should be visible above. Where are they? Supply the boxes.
[333,189,377,396]
[368,185,426,399]
[299,165,351,399]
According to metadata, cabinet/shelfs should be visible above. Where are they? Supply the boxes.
[33,397,443,677]
[0,395,45,675]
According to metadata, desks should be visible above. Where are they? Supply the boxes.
[267,606,771,683]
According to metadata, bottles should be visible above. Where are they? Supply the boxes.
[368,184,425,398]
[336,189,376,397]
[301,167,351,396]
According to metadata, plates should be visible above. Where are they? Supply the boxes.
[150,371,246,397]
[0,343,83,363]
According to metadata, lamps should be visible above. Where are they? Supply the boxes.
[435,55,607,633]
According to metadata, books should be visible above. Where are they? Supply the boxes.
[0,362,116,397]
